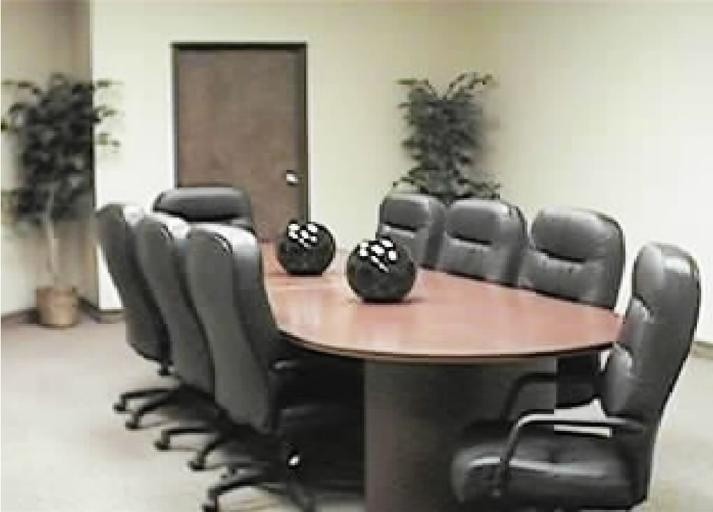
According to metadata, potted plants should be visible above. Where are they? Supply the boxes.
[0,74,123,328]
[397,69,504,200]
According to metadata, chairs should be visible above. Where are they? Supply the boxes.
[93,201,212,431]
[181,223,359,510]
[450,240,699,509]
[516,206,626,408]
[435,198,527,287]
[133,213,269,473]
[153,186,256,236]
[376,184,447,273]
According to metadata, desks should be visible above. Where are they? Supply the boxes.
[258,242,624,508]
